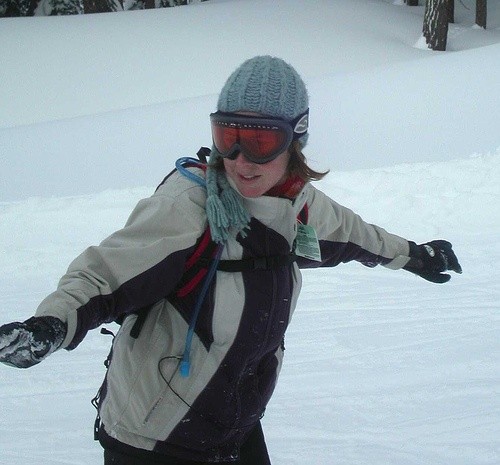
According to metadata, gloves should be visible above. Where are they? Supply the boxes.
[402,240,462,283]
[0,316,67,368]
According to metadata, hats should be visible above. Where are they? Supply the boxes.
[206,55,309,244]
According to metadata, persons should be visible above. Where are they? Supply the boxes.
[1,52,463,464]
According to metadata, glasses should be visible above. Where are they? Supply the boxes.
[210,109,308,164]
